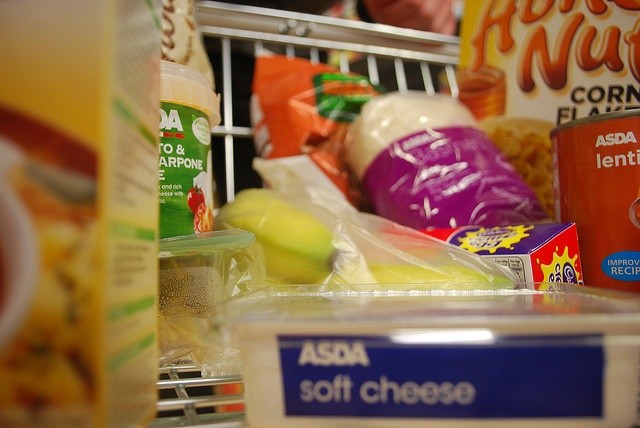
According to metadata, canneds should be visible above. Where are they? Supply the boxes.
[549,110,640,294]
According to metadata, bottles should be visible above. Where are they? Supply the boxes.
[159,60,222,239]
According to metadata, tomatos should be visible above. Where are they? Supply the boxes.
[186,183,205,214]
